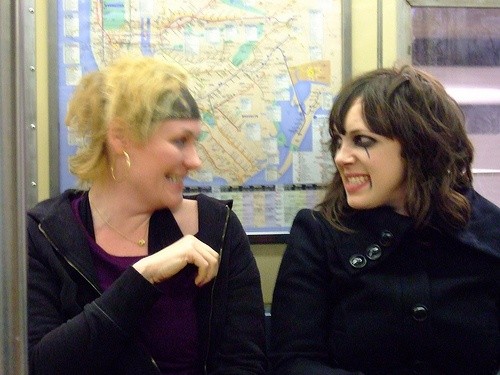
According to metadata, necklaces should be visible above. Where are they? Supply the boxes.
[88,196,148,247]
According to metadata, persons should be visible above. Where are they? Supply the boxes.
[25,57,268,375]
[268,65,500,374]
